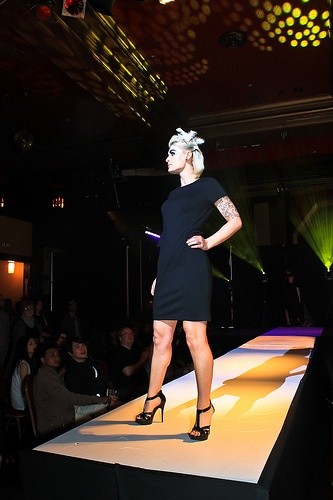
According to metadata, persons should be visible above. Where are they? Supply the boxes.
[134,128,243,441]
[0,290,199,471]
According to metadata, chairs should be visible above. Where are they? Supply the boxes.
[0,365,38,440]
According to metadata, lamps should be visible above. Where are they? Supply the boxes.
[59,0,87,19]
[14,87,34,154]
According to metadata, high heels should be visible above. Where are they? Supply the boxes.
[188,400,215,441]
[135,390,166,425]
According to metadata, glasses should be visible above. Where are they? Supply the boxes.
[121,332,135,336]
